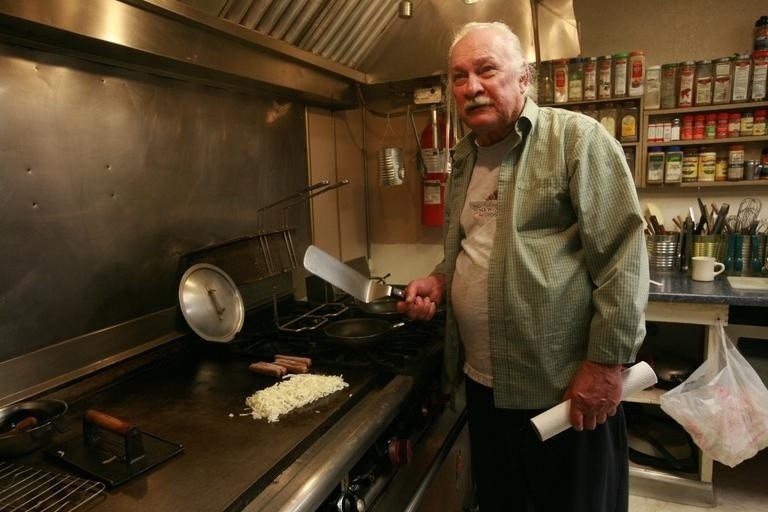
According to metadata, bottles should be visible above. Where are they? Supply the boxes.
[623,147,634,181]
[647,145,744,184]
[569,100,640,143]
[537,51,645,106]
[644,15,767,110]
[647,110,768,143]
[760,149,768,179]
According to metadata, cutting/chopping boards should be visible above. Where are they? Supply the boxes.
[727,276,768,290]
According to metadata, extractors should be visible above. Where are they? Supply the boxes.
[0,0,412,108]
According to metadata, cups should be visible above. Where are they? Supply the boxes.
[691,255,727,283]
[745,160,763,180]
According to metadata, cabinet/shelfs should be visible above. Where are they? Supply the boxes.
[617,318,714,505]
[643,101,768,190]
[537,99,642,187]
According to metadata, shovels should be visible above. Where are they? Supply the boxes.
[303,245,405,304]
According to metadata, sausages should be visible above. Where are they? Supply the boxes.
[248,354,312,381]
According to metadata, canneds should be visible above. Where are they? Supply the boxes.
[714,156,728,182]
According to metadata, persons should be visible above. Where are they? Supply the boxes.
[393,21,650,512]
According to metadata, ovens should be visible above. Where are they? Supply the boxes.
[363,377,480,511]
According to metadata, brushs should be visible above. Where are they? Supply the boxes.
[644,208,655,235]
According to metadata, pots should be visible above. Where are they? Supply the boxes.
[323,316,391,344]
[655,359,702,390]
[361,298,407,317]
[626,411,693,468]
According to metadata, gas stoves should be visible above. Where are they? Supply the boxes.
[198,283,447,374]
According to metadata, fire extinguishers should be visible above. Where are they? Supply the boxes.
[390,78,453,227]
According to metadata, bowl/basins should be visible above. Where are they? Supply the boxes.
[0,399,68,455]
